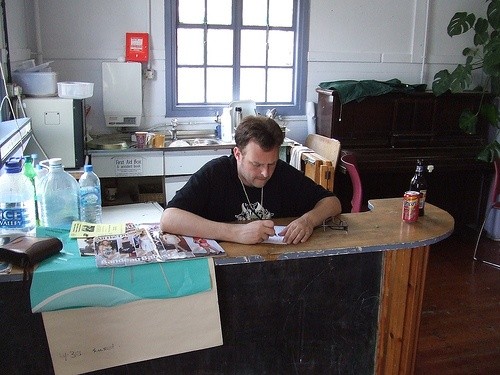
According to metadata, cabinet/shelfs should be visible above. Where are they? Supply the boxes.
[315,88,494,170]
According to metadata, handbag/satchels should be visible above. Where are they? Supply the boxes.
[0,236,63,281]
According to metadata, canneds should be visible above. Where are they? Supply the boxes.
[402,191,420,222]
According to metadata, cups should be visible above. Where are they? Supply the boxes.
[134,132,149,143]
[152,134,165,149]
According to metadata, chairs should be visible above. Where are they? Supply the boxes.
[340,154,370,214]
[471,150,500,266]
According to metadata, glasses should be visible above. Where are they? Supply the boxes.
[321,215,348,232]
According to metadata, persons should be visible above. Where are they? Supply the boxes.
[159,115,341,246]
[98,240,115,267]
[162,232,188,254]
[119,237,135,258]
[193,237,222,257]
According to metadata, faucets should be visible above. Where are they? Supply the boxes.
[166,119,178,138]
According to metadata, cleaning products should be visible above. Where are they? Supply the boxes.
[220,106,233,143]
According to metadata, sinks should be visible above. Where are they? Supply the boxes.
[164,138,219,148]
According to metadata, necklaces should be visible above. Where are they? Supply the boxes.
[239,177,263,211]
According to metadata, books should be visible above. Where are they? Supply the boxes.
[76,223,226,269]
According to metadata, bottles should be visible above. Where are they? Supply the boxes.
[408,158,428,216]
[220,106,234,142]
[22,157,36,185]
[79,165,102,225]
[36,157,78,229]
[0,156,36,240]
[234,107,242,129]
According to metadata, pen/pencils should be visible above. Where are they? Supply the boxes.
[248,207,278,237]
